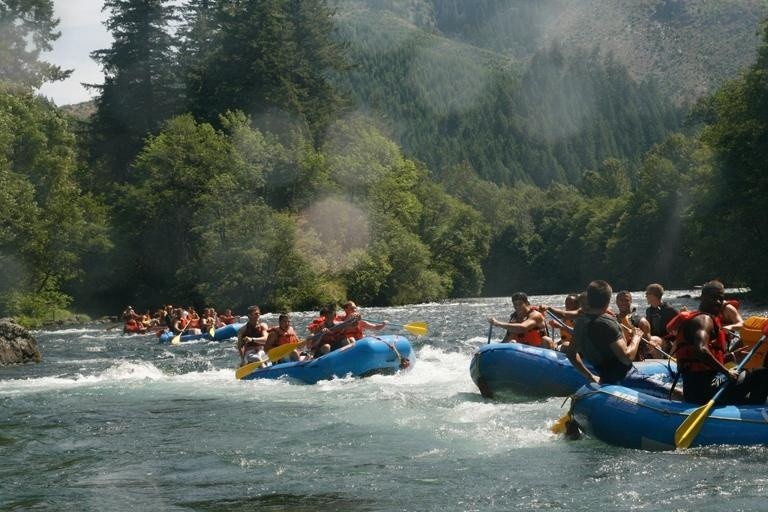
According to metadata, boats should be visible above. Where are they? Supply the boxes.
[238,334,418,386]
[157,320,246,344]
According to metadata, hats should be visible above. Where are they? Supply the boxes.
[512,291,531,305]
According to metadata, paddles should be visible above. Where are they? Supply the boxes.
[268,314,361,363]
[171,320,191,345]
[210,311,217,338]
[142,318,157,325]
[674,334,766,453]
[236,358,270,379]
[361,318,428,336]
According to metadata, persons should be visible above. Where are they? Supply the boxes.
[122,301,389,368]
[488,280,768,406]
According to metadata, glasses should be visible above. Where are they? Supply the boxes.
[646,292,654,296]
